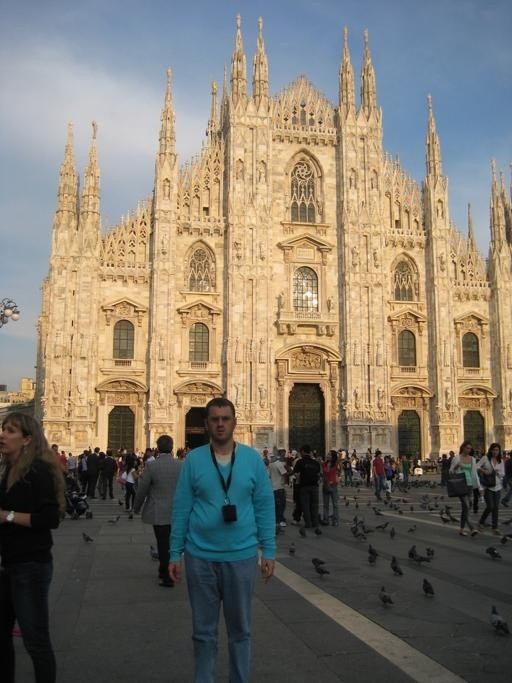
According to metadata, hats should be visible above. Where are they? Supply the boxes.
[374,451,381,457]
[298,445,313,451]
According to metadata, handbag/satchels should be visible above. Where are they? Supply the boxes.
[480,470,496,487]
[447,473,466,497]
[118,477,127,484]
[324,481,337,494]
[383,479,392,494]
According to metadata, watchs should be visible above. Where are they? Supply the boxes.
[6,510,14,522]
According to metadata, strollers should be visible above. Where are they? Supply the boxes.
[63,478,93,520]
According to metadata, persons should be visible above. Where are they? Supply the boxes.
[474,442,505,536]
[288,445,321,528]
[262,444,419,502]
[270,456,286,526]
[319,450,339,527]
[177,447,192,460]
[448,440,485,538]
[0,409,69,682]
[440,449,511,507]
[166,396,279,683]
[51,442,159,520]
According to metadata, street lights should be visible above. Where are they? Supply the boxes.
[0,298,20,328]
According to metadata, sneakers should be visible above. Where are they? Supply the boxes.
[292,520,298,525]
[279,521,287,527]
